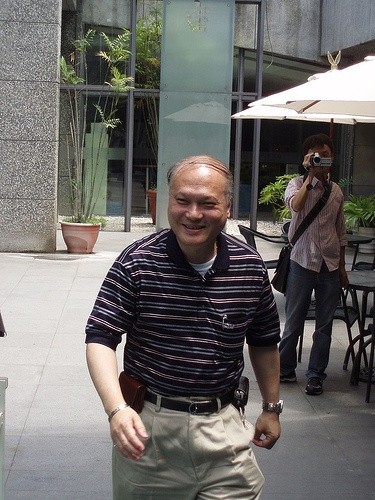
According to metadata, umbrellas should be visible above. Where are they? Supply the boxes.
[230,55,375,157]
[165,103,232,127]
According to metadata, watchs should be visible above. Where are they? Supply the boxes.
[263,401,284,416]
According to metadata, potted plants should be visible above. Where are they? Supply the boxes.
[134,5,164,225]
[59,29,134,253]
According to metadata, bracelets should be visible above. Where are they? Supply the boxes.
[107,404,130,424]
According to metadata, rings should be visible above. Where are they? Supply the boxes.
[114,442,119,448]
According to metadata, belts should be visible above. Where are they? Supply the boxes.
[143,393,232,415]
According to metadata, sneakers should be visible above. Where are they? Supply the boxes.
[281,369,298,383]
[305,377,323,396]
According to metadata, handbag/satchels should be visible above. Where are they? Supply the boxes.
[268,245,290,295]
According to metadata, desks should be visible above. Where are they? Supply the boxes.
[342,234,373,300]
[339,270,375,387]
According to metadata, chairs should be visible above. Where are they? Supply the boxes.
[238,221,375,363]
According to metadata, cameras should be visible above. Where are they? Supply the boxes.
[313,152,331,167]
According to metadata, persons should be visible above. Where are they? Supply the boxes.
[277,135,349,396]
[85,155,282,500]
